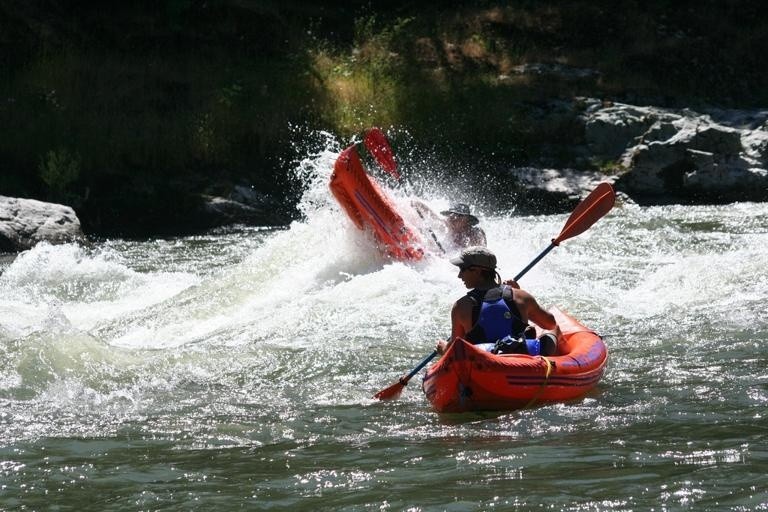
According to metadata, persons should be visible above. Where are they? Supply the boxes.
[433,246,559,358]
[410,200,487,248]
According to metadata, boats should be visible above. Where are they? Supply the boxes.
[324,139,426,266]
[419,299,612,415]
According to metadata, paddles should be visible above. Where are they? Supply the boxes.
[372,183,615,401]
[365,129,445,254]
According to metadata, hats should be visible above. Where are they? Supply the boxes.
[449,246,498,269]
[439,202,479,226]
